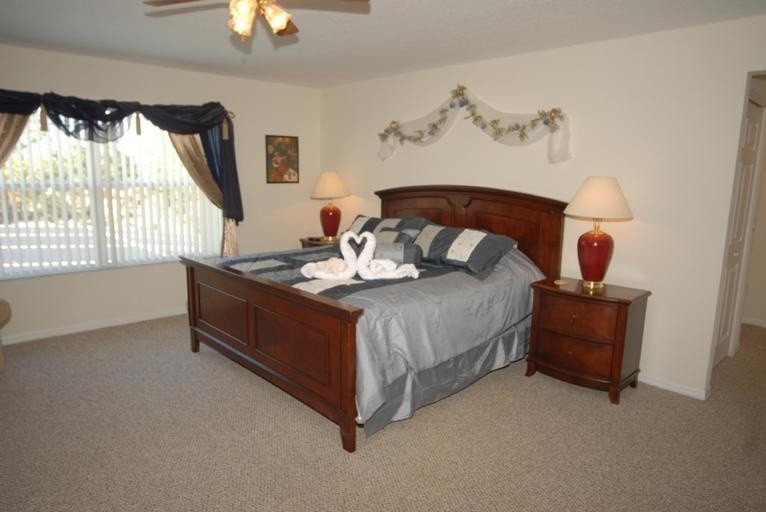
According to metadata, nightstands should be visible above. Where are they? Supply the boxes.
[525,275,653,405]
[299,238,341,249]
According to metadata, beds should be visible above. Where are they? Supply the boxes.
[178,183,569,453]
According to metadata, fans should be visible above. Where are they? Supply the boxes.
[141,1,370,19]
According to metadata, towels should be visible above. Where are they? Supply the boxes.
[300,230,420,281]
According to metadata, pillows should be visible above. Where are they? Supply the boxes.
[340,214,518,282]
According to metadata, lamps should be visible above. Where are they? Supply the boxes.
[310,170,351,240]
[226,1,298,42]
[563,175,634,289]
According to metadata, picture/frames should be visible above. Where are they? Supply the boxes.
[265,135,300,184]
